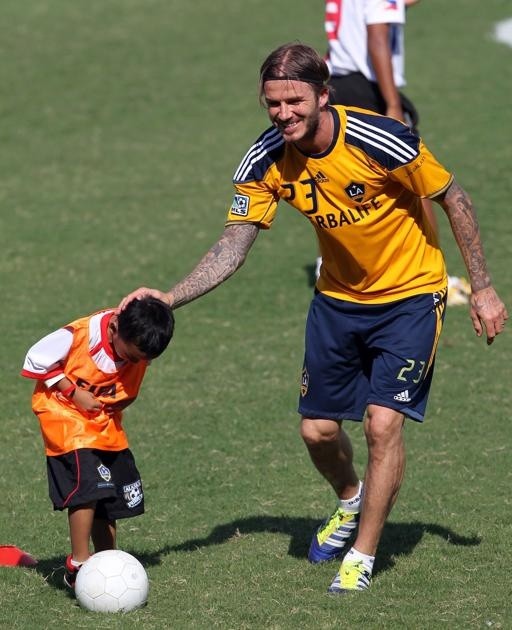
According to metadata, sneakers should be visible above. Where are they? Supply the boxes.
[328,548,375,593]
[62,555,83,589]
[307,506,359,564]
[446,278,475,307]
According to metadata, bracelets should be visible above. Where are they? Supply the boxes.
[62,384,76,397]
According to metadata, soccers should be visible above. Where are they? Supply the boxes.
[74,549,149,614]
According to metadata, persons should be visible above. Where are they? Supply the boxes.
[316,0,471,306]
[20,297,175,597]
[114,39,509,593]
